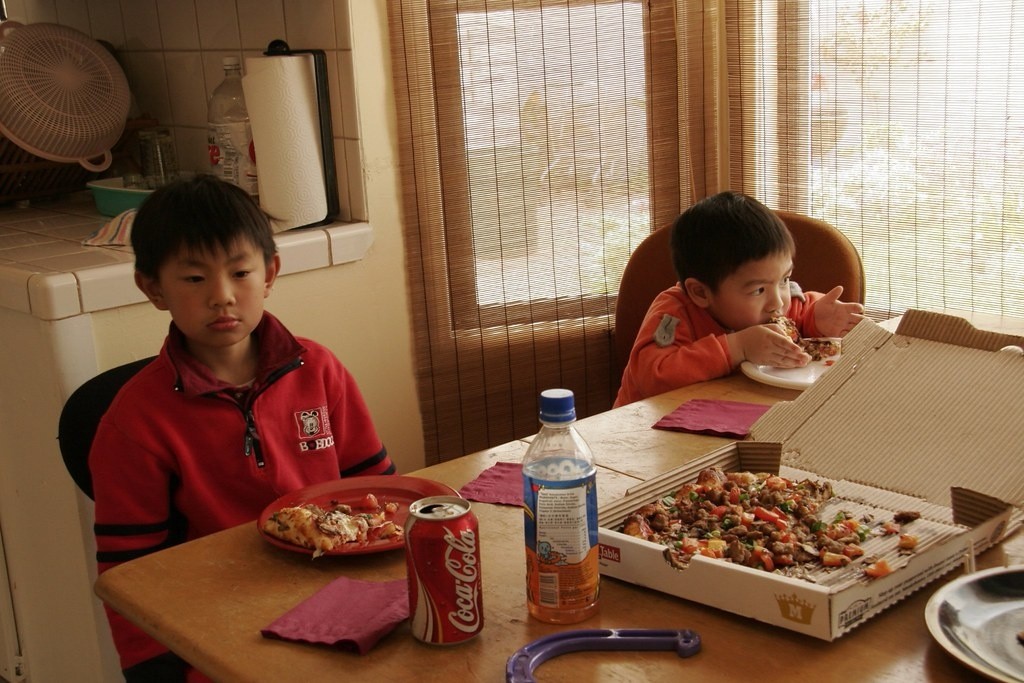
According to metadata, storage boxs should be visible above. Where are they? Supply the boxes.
[602,307,1024,643]
[85,175,154,216]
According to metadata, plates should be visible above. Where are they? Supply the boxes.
[924,562,1024,683]
[740,337,842,390]
[257,476,461,555]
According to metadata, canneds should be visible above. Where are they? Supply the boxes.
[403,495,483,647]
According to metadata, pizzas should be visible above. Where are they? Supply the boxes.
[772,315,840,362]
[619,466,862,569]
[261,505,384,556]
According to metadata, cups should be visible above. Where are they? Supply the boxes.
[124,174,141,190]
[138,128,180,190]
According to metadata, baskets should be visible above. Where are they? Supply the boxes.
[0,21,131,173]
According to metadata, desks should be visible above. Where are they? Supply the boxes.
[100,378,1024,683]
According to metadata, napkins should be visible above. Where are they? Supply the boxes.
[458,462,524,508]
[262,576,411,653]
[653,398,772,438]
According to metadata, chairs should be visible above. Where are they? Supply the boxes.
[54,354,157,500]
[617,210,868,384]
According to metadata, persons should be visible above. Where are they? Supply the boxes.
[612,190,869,409]
[88,173,397,683]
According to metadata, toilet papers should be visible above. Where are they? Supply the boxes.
[241,53,327,234]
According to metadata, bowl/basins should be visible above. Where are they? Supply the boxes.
[86,171,191,217]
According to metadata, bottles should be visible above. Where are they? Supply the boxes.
[522,389,600,624]
[206,56,259,199]
